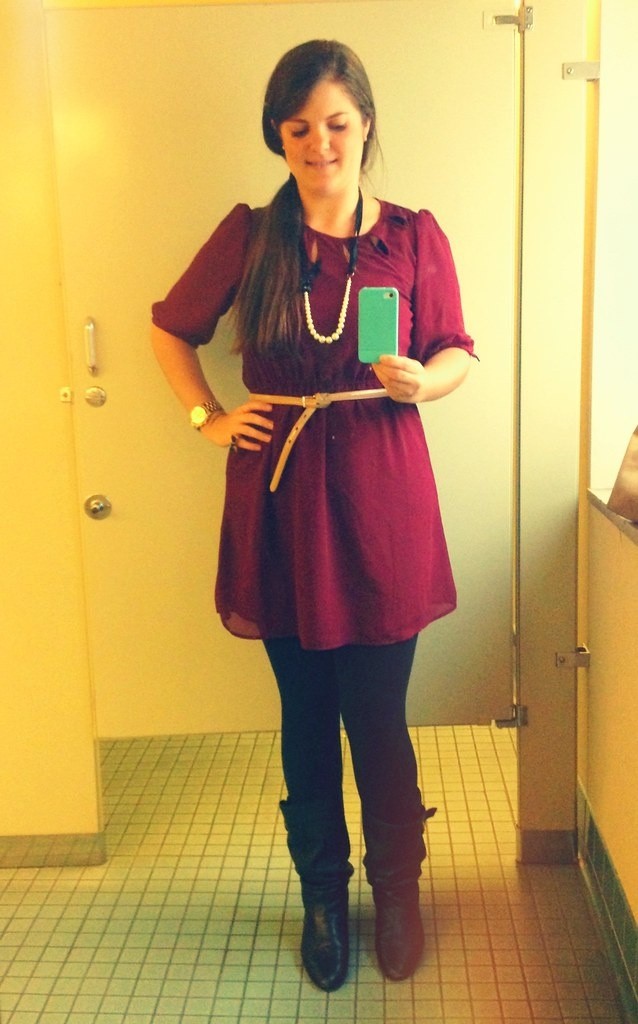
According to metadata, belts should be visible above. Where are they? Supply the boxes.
[247,388,389,492]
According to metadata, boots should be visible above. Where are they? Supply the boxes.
[362,787,436,980]
[282,796,353,992]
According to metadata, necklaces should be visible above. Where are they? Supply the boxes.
[299,186,362,343]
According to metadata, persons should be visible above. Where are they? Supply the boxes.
[151,40,478,993]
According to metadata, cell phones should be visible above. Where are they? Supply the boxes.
[357,286,400,365]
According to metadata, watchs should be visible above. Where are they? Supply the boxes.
[190,401,223,431]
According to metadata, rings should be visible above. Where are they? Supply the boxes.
[231,434,246,448]
